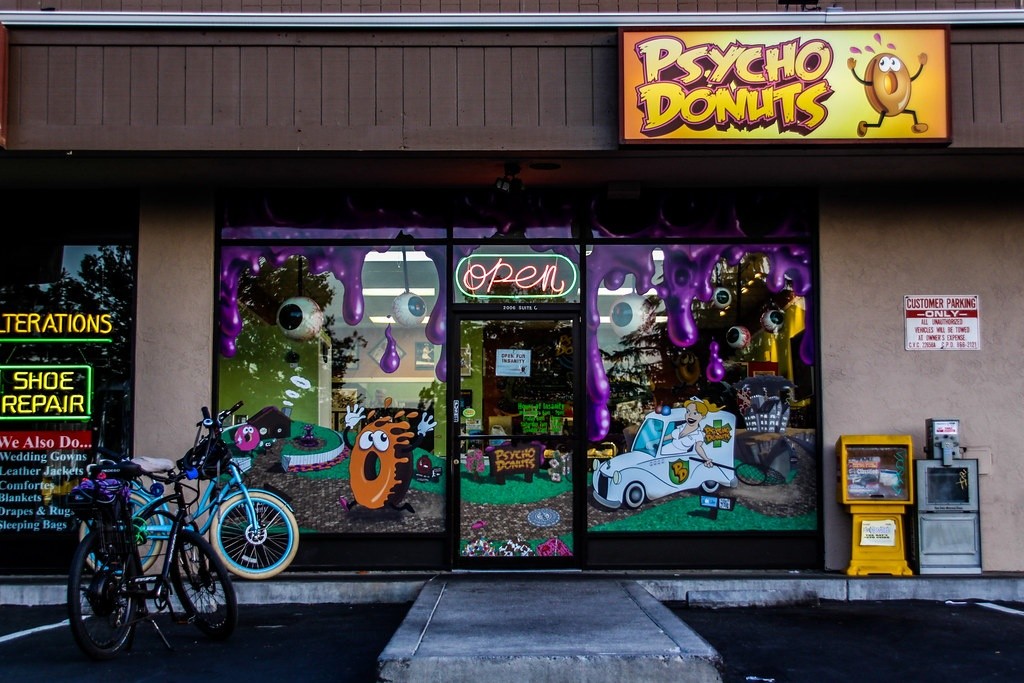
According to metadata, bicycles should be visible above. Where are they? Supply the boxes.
[56,400,300,663]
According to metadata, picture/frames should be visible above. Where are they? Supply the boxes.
[460,346,471,376]
[342,338,360,370]
